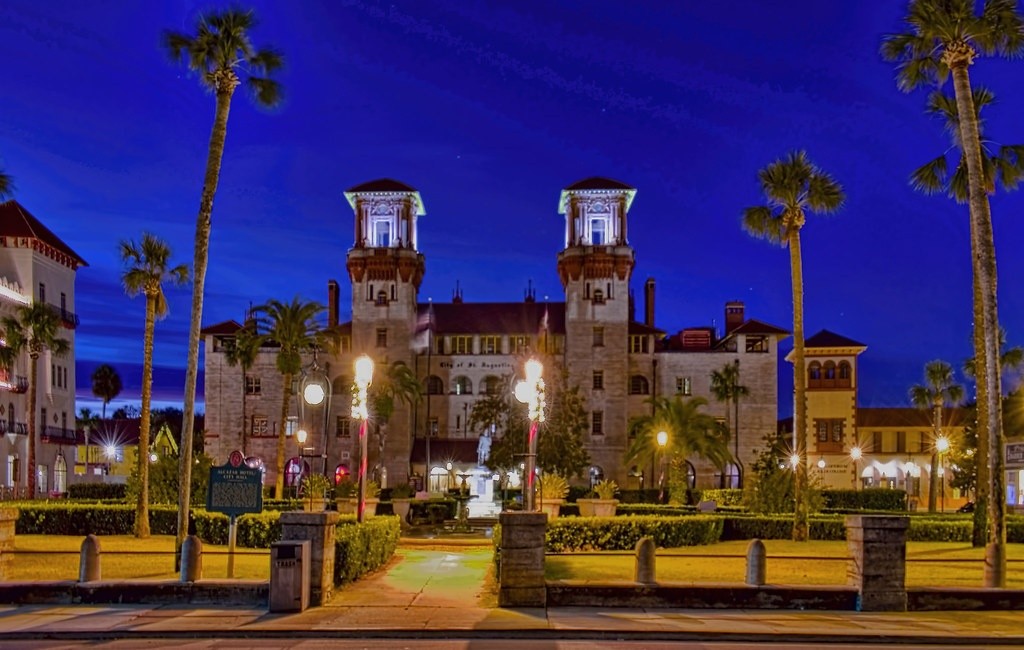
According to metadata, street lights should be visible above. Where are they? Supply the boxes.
[935,436,949,513]
[349,352,375,523]
[657,431,669,505]
[513,358,546,513]
[850,447,862,491]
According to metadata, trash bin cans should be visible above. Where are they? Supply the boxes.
[269,540,311,613]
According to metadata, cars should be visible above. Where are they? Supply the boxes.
[958,500,975,513]
